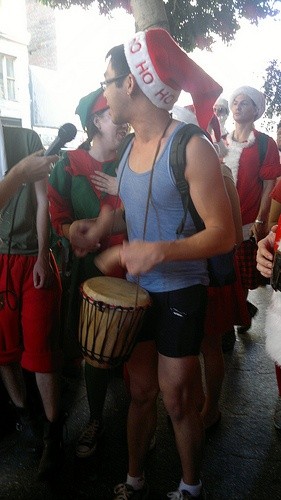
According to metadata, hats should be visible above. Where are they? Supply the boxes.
[228,86,266,121]
[173,103,223,144]
[124,27,223,130]
[90,93,108,114]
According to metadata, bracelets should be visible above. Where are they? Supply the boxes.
[255,220,265,224]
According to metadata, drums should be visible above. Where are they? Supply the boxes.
[78,275,153,369]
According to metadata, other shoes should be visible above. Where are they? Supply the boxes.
[112,484,137,500]
[237,301,259,333]
[274,396,281,428]
[1,402,30,436]
[76,426,97,454]
[36,412,63,482]
[164,487,206,499]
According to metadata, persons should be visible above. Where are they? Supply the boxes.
[100,30,236,500]
[220,87,281,332]
[174,104,239,434]
[0,114,66,484]
[46,87,129,459]
[257,122,281,430]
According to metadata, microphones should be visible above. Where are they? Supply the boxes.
[42,123,77,157]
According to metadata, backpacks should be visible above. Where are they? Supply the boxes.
[115,122,237,288]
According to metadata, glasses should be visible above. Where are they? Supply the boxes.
[100,75,123,89]
